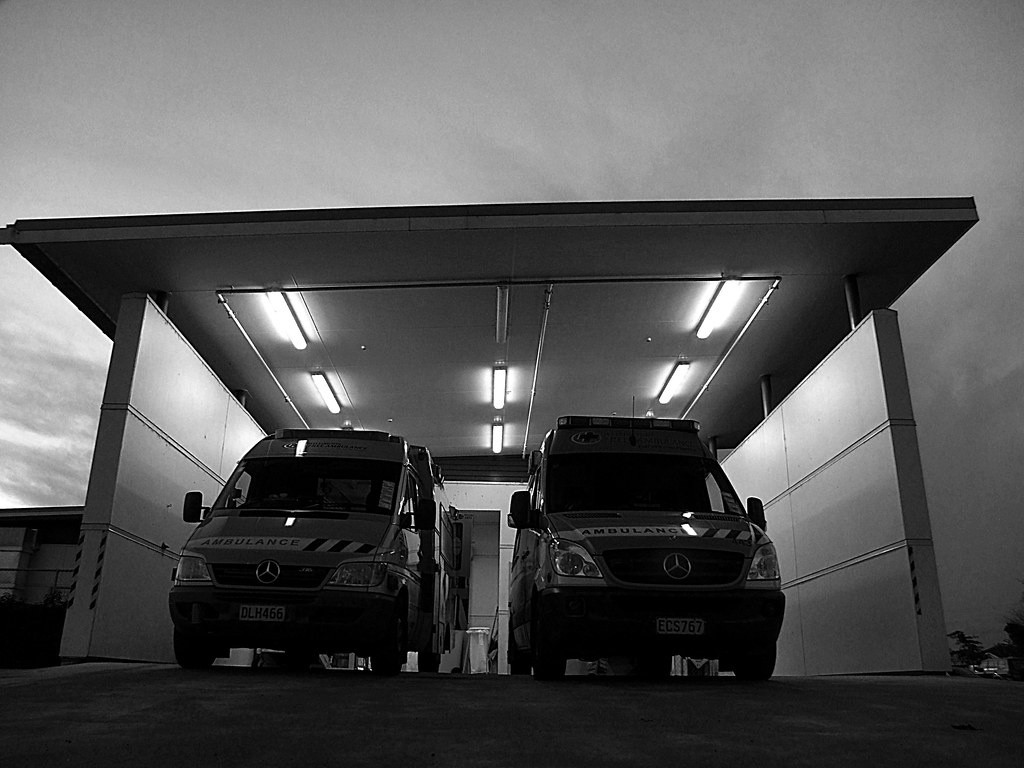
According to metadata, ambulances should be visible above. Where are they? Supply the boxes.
[508,414,787,688]
[163,419,461,679]
